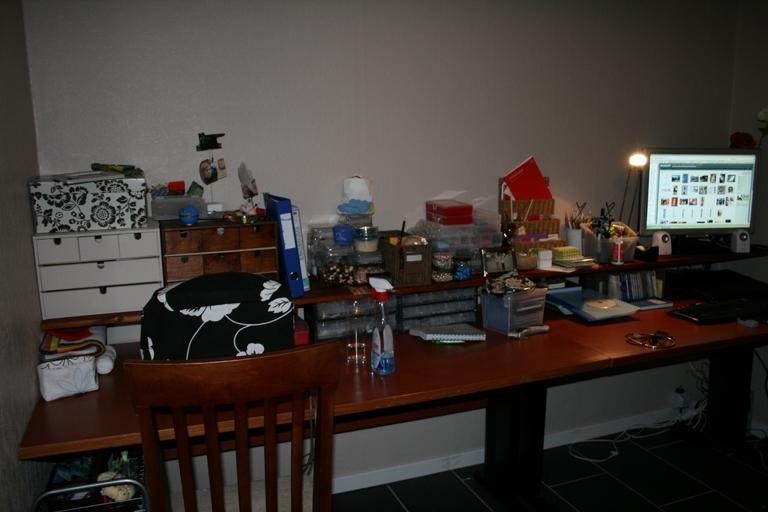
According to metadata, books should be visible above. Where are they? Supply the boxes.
[411,323,486,341]
[502,156,553,200]
[609,270,663,300]
[50,169,125,185]
[629,299,674,310]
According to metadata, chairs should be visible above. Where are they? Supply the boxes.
[123,336,342,512]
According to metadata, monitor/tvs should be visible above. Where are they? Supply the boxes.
[639,147,762,236]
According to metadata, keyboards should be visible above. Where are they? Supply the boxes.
[667,296,768,325]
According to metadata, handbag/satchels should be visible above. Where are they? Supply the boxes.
[139,270,296,361]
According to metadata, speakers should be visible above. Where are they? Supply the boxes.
[652,231,672,256]
[731,230,750,254]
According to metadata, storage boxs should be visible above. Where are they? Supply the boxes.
[26,171,148,234]
[151,193,207,220]
[480,286,548,335]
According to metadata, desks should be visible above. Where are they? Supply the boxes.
[18,243,768,511]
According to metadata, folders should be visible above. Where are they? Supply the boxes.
[290,203,311,293]
[263,191,306,300]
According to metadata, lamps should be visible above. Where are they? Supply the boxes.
[619,152,648,237]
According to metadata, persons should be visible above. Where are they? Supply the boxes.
[199,162,218,184]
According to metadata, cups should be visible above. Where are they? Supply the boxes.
[346,305,368,369]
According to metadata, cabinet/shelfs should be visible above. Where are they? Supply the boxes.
[162,219,280,282]
[31,216,164,320]
[31,459,151,512]
